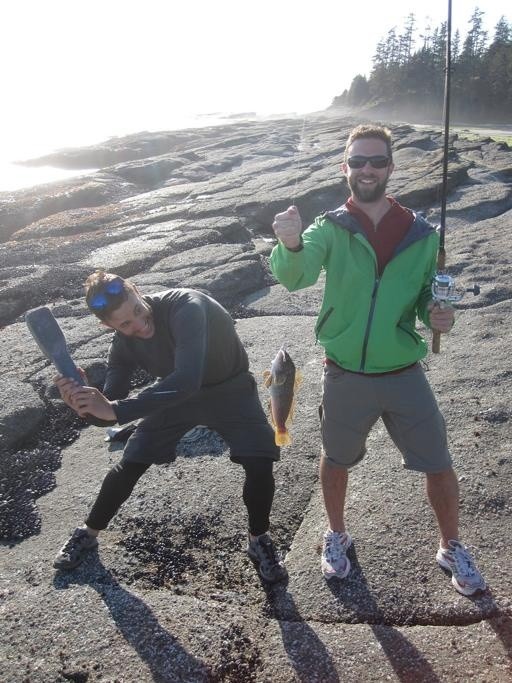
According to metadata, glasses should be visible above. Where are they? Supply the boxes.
[347,156,390,168]
[87,276,125,310]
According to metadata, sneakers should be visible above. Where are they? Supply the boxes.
[247,528,287,582]
[436,537,487,596]
[321,524,353,581]
[53,528,99,571]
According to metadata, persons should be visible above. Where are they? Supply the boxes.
[51,272,288,581]
[268,125,487,599]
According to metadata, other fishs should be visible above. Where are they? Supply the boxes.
[261,348,302,448]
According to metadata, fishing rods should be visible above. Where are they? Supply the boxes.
[431,0,480,354]
